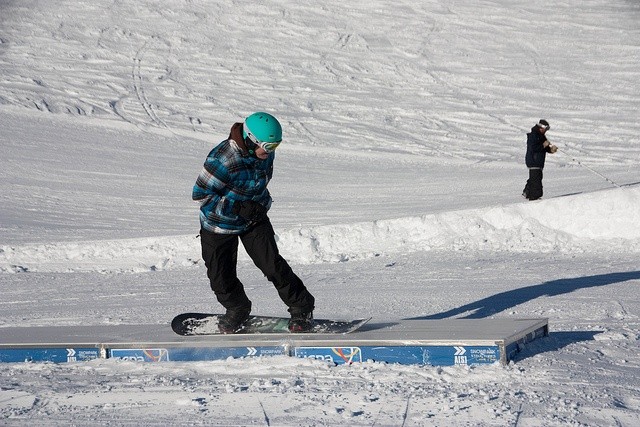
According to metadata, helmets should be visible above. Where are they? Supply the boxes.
[243,112,282,153]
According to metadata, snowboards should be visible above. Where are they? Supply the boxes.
[170,312,372,337]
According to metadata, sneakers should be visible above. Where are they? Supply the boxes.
[218,302,252,332]
[287,305,313,331]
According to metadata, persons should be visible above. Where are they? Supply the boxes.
[521,118,558,201]
[191,112,314,332]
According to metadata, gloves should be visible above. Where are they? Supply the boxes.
[544,140,550,148]
[232,199,266,221]
[551,145,558,152]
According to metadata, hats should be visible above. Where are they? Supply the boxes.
[539,120,549,128]
